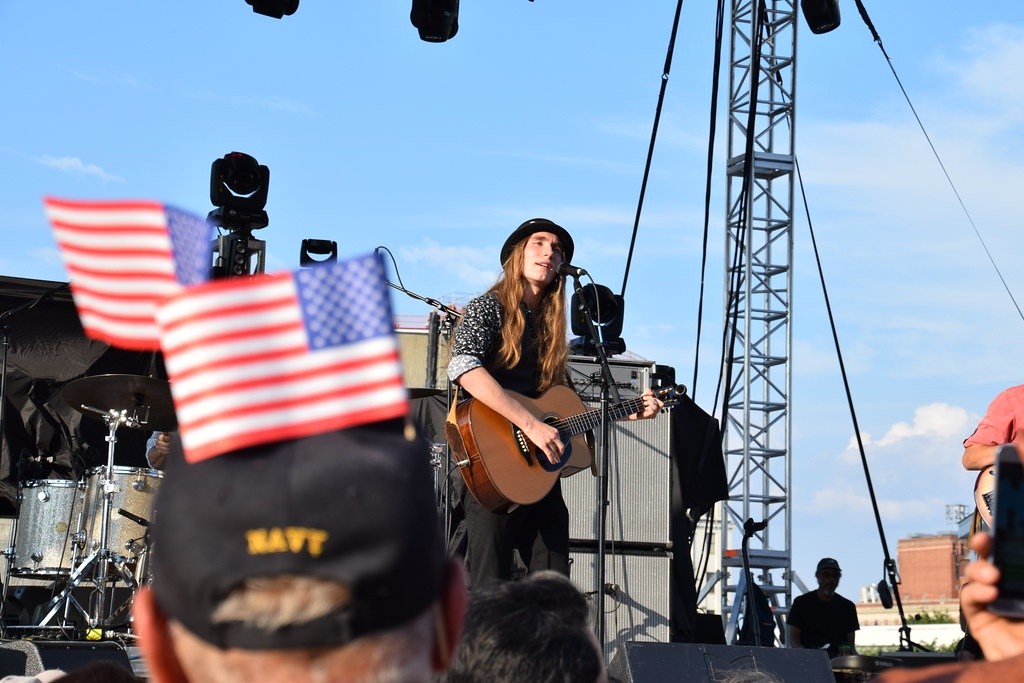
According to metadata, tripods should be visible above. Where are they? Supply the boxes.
[40,404,140,642]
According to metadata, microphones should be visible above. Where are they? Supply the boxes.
[604,583,619,594]
[556,262,587,278]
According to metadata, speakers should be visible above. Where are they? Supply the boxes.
[607,641,837,683]
[568,547,673,683]
[0,639,133,678]
[560,401,673,545]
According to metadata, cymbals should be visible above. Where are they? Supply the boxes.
[62,370,175,418]
[830,653,904,676]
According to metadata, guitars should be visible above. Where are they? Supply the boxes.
[445,384,681,518]
[740,518,775,648]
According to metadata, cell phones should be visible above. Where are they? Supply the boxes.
[986,444,1024,620]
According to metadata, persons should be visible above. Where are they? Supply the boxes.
[145,430,171,470]
[787,559,861,655]
[958,532,1024,663]
[445,219,664,587]
[961,384,1024,471]
[131,432,449,683]
[434,571,609,683]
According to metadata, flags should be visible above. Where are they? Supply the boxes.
[43,196,213,350]
[155,253,410,464]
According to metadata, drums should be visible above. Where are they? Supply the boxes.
[80,465,168,581]
[0,515,60,589]
[11,477,85,582]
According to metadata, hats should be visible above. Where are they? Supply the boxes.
[500,218,574,270]
[815,558,842,575]
[148,432,447,650]
